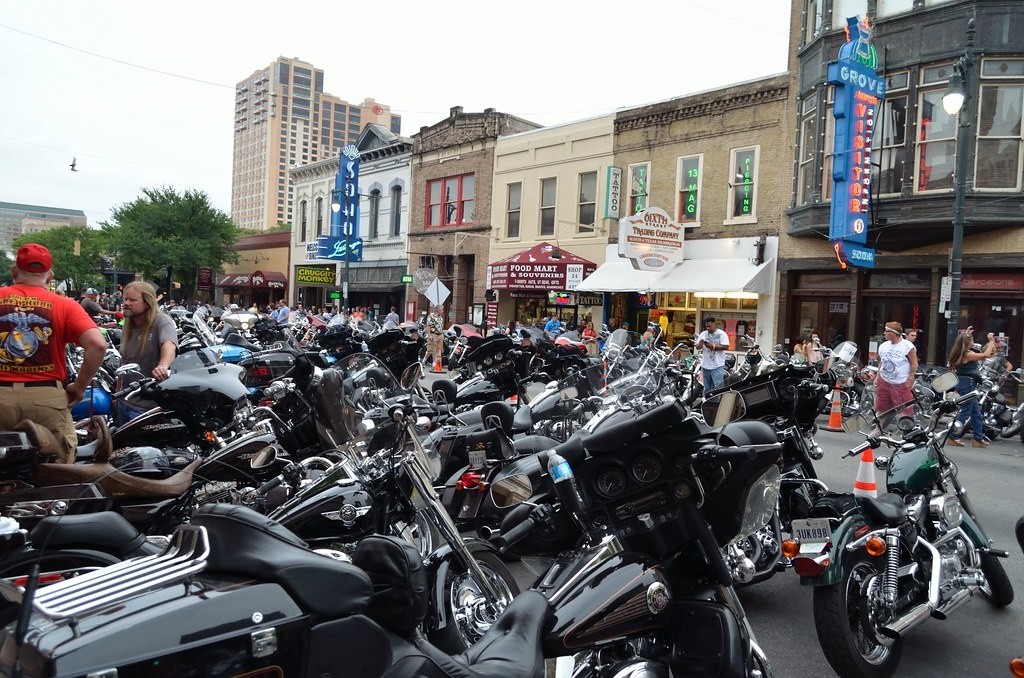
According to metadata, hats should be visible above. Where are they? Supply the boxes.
[16,244,52,272]
[86,288,100,294]
[885,321,902,335]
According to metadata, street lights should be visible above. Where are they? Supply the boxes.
[943,60,970,366]
[330,189,350,314]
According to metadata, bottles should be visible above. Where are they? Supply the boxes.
[547,450,585,514]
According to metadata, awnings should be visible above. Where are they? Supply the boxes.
[215,270,288,290]
[486,243,595,292]
[574,258,774,294]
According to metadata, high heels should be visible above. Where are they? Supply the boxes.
[947,436,965,446]
[972,440,988,447]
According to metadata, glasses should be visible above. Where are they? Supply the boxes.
[885,331,892,332]
[964,331,969,337]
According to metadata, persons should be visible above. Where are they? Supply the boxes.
[868,321,918,440]
[903,329,916,342]
[947,325,996,446]
[506,320,517,338]
[111,280,177,409]
[983,344,1013,376]
[694,318,730,400]
[544,315,664,347]
[422,304,443,368]
[481,313,489,338]
[792,331,823,365]
[0,244,107,464]
[76,286,399,327]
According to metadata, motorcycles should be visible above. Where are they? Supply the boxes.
[1,293,1024,678]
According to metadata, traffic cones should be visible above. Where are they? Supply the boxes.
[820,382,847,429]
[509,394,517,405]
[430,343,447,372]
[850,447,878,499]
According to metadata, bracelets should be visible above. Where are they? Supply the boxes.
[712,344,715,350]
[907,376,914,380]
